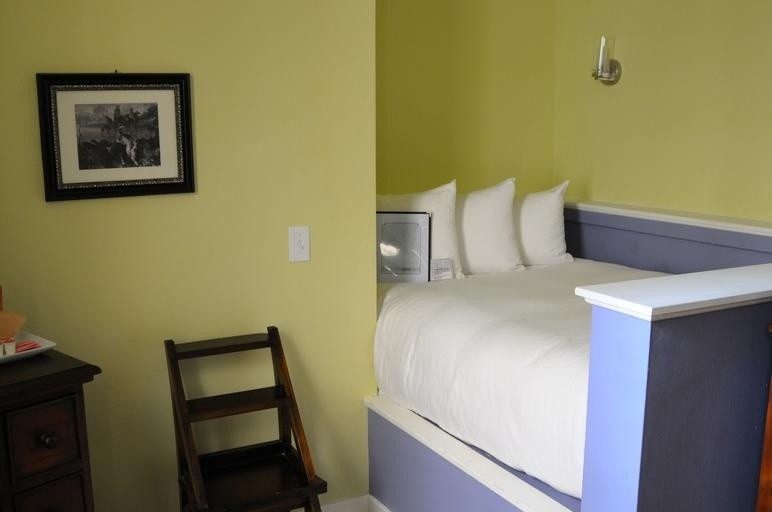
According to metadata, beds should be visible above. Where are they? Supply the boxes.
[363,200,772,511]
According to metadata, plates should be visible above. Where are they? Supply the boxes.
[0,329,57,363]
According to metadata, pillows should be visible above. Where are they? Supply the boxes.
[375,178,575,281]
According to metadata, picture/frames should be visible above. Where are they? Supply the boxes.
[37,72,195,202]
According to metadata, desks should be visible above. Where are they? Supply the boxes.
[0,348,102,512]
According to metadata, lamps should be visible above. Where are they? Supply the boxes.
[590,33,622,86]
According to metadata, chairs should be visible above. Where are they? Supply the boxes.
[163,326,328,512]
